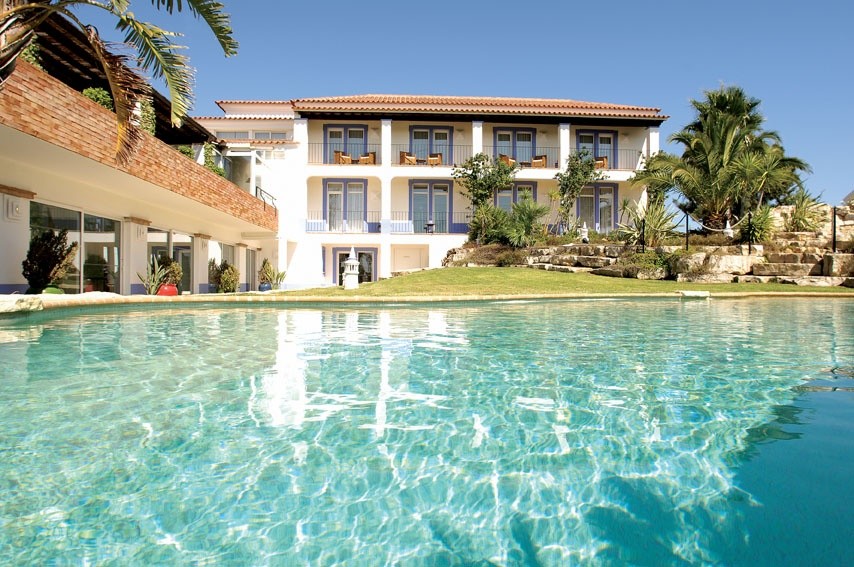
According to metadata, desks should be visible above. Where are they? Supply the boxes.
[520,162,531,169]
[416,159,426,165]
[351,159,359,163]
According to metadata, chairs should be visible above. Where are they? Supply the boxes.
[333,150,353,164]
[359,152,376,164]
[424,223,436,235]
[594,156,608,168]
[499,154,516,168]
[400,151,416,165]
[531,155,547,167]
[427,153,442,165]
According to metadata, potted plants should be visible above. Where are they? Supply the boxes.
[152,256,182,295]
[257,258,271,290]
[22,229,78,294]
[207,258,241,293]
[264,270,287,290]
